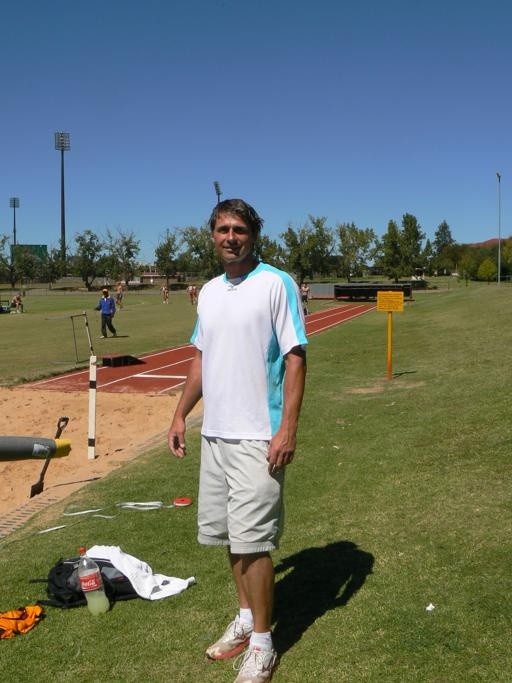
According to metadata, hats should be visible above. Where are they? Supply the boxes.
[103,289,108,292]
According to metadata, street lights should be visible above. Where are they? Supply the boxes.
[9,195,21,243]
[496,171,501,283]
[54,128,73,274]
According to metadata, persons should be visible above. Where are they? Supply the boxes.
[167,199,312,683]
[10,293,26,314]
[95,282,124,338]
[160,284,169,305]
[187,284,198,305]
[299,282,310,315]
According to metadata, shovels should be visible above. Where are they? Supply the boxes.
[30,417,70,498]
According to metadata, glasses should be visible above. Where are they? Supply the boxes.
[0,608,26,620]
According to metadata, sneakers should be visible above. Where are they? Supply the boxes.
[205,615,277,683]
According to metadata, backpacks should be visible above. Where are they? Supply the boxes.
[29,557,137,608]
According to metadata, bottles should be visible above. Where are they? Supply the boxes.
[77,546,110,616]
